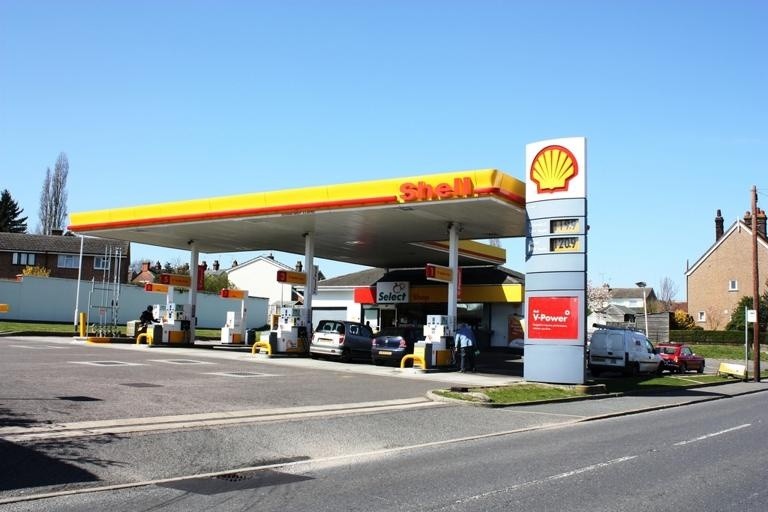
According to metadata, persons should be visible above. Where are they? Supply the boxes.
[140,305,160,327]
[454,322,478,374]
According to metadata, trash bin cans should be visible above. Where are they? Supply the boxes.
[247,330,256,345]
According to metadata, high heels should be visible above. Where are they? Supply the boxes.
[458,369,465,373]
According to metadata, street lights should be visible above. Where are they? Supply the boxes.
[634,281,648,338]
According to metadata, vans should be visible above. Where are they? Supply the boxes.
[308,319,374,362]
[587,323,664,379]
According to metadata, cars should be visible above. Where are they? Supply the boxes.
[654,342,705,374]
[370,327,425,365]
